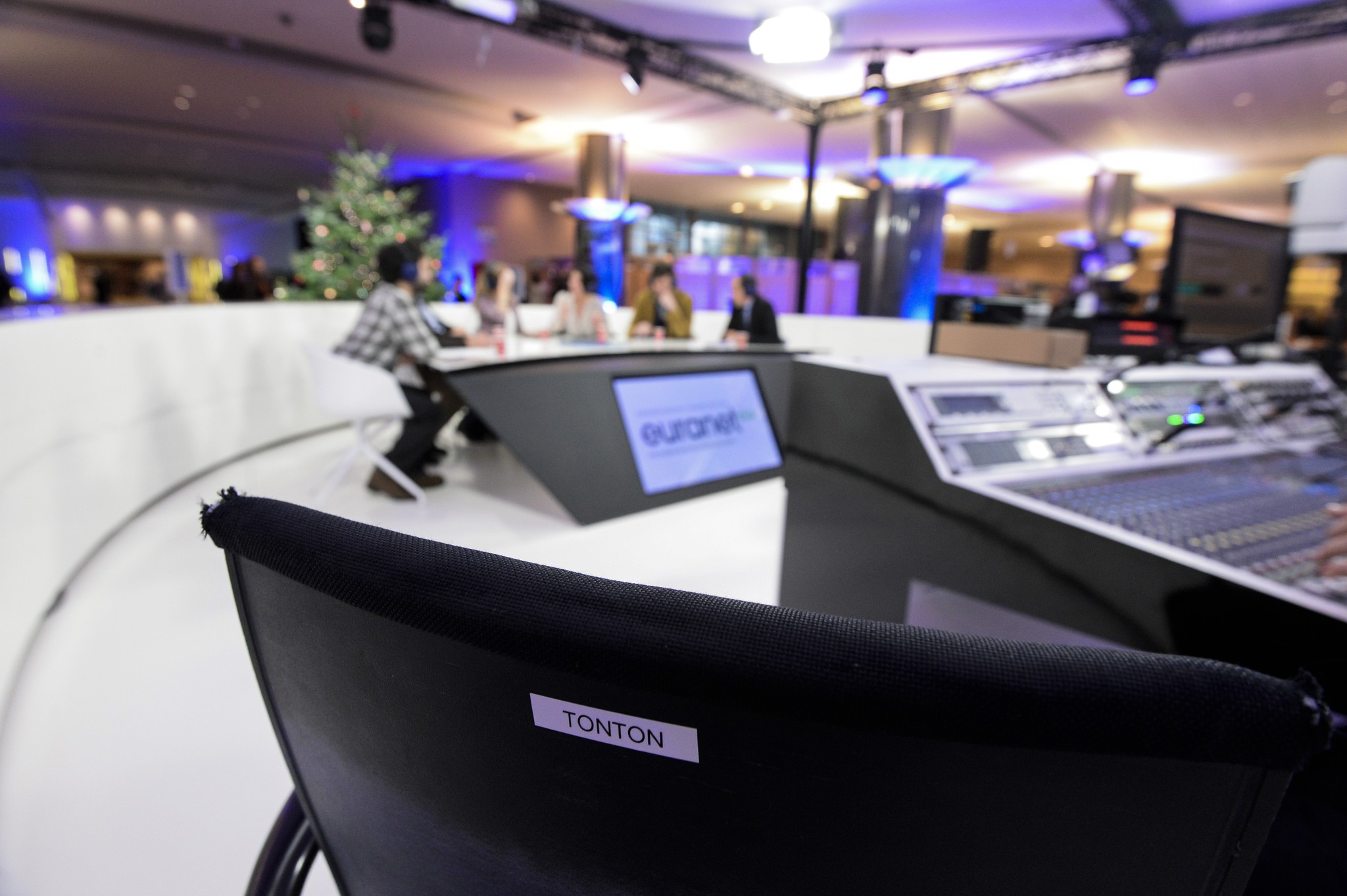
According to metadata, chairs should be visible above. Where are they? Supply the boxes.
[198,485,1346,896]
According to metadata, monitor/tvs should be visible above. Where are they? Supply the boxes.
[613,367,783,496]
[1160,204,1292,353]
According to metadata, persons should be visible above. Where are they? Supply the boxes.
[89,252,316,303]
[332,240,446,501]
[553,265,614,338]
[628,260,693,340]
[719,273,785,344]
[399,241,492,465]
[453,259,523,446]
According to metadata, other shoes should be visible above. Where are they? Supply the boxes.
[411,474,445,487]
[422,445,445,464]
[369,468,413,499]
[457,419,498,443]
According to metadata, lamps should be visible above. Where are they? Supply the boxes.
[619,42,652,96]
[1123,34,1158,98]
[863,58,889,105]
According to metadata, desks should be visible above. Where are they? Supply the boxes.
[422,337,815,527]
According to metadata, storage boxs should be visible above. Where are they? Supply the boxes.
[934,319,1091,371]
